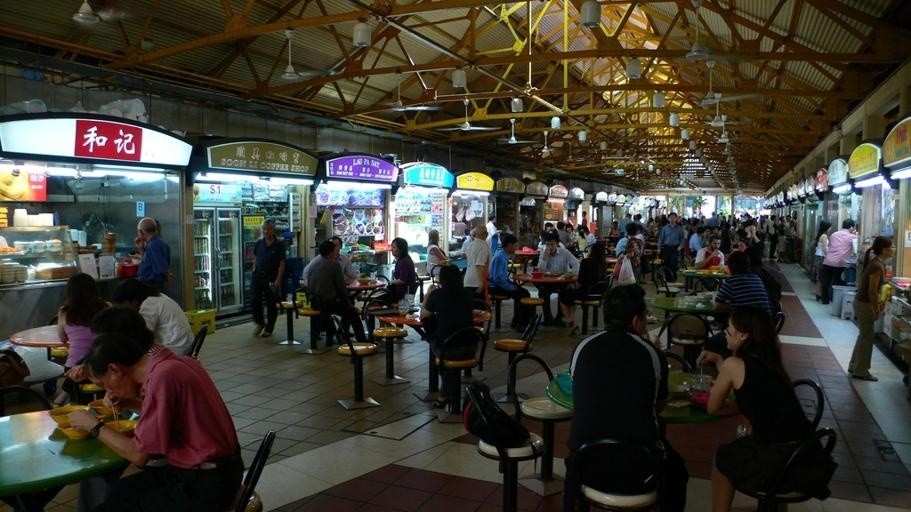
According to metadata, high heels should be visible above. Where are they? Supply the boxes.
[434,398,451,413]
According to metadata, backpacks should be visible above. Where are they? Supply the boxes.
[625,236,644,268]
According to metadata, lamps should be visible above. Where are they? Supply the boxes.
[581,1,727,192]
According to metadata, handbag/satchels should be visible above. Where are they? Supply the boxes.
[0,346,30,386]
[877,283,892,313]
[462,381,529,448]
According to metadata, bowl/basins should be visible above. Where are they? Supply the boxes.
[101,232,116,253]
[532,272,544,280]
[357,279,370,284]
[48,400,139,440]
[685,296,704,304]
[667,374,719,407]
[0,263,28,283]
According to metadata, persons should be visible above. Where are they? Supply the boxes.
[847,235,897,382]
[54,272,113,405]
[822,218,858,303]
[300,210,798,405]
[697,302,839,512]
[561,283,689,511]
[134,217,172,292]
[67,330,246,511]
[249,218,287,337]
[814,221,832,301]
[111,276,195,357]
[64,304,164,400]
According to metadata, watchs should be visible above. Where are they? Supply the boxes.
[90,422,104,438]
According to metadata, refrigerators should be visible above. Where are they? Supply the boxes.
[189,182,241,309]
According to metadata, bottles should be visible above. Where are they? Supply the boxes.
[195,211,210,303]
[218,211,233,305]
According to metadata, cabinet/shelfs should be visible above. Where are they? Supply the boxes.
[0,226,79,340]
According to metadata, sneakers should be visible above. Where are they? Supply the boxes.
[852,373,878,381]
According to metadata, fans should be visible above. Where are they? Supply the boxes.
[646,0,756,197]
[70,1,137,27]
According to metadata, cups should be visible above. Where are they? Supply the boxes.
[405,294,414,308]
